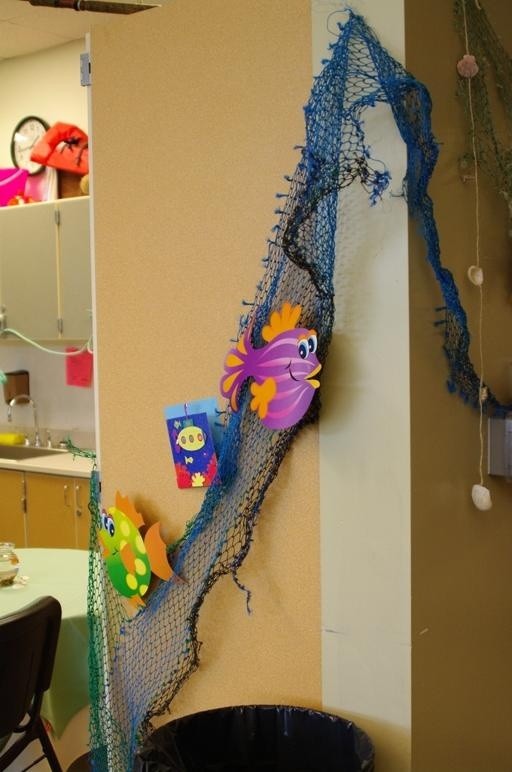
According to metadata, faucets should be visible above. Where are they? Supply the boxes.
[7,393,40,447]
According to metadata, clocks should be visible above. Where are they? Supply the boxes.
[10,114,50,176]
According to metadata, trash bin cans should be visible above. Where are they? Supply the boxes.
[134,705,375,772]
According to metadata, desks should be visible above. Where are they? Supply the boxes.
[0,547,106,772]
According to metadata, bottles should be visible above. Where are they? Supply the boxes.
[1,542,20,586]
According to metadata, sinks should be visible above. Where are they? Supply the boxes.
[0,444,70,461]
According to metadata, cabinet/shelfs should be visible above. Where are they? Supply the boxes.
[0,468,26,548]
[23,471,90,551]
[0,196,61,342]
[56,195,92,340]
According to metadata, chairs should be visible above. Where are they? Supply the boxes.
[0,595,63,771]
